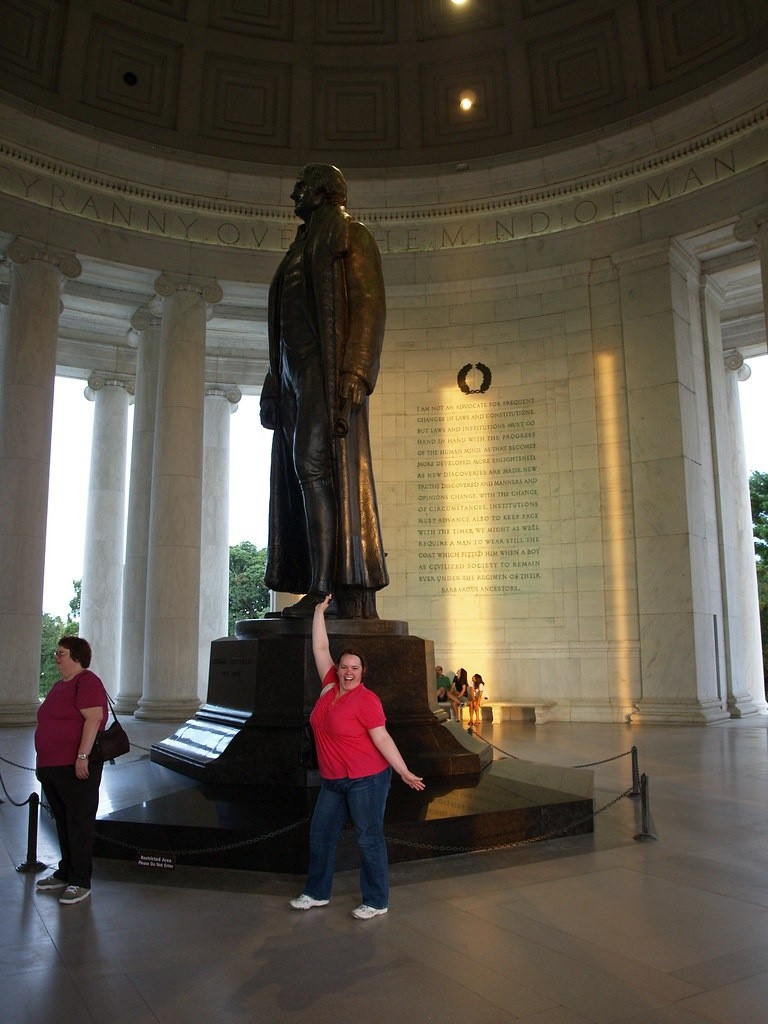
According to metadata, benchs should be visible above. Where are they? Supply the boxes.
[438,699,558,725]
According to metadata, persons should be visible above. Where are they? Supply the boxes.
[436,666,451,702]
[468,674,489,726]
[447,668,469,722]
[288,593,425,920]
[34,635,109,904]
[260,165,390,616]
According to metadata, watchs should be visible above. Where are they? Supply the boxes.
[77,754,88,760]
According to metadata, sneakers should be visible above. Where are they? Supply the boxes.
[59,885,91,904]
[36,875,69,889]
[352,904,388,920]
[288,895,329,909]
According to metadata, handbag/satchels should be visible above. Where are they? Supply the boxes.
[76,672,130,764]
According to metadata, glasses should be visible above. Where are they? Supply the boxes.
[54,651,70,657]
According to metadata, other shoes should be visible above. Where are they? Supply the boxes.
[468,722,473,725]
[474,722,480,725]
[456,720,461,723]
[459,703,466,709]
